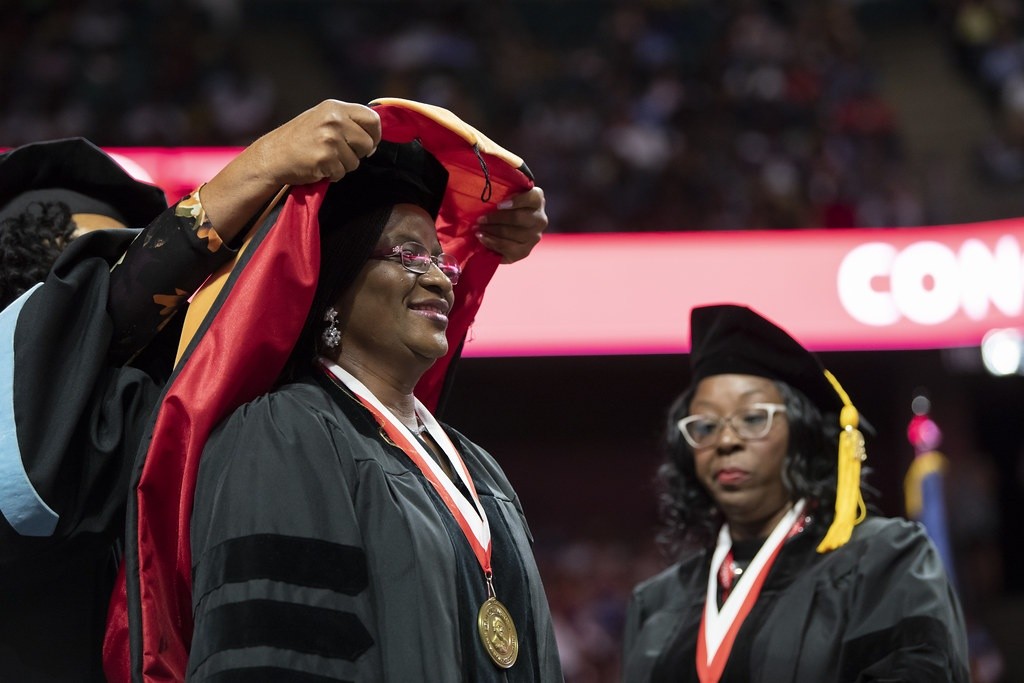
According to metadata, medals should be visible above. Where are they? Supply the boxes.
[479,597,519,669]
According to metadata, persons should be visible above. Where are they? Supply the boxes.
[0,99,549,683]
[620,304,972,683]
[186,139,563,683]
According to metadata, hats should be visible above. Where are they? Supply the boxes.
[310,139,450,236]
[0,137,169,231]
[683,303,873,554]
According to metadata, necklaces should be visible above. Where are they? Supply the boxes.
[409,425,426,435]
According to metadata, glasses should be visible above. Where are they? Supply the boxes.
[676,403,787,449]
[371,241,463,286]
